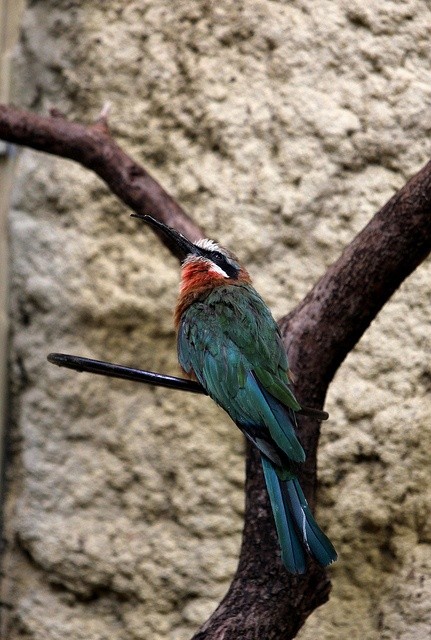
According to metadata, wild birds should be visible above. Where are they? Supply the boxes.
[129,211,339,576]
